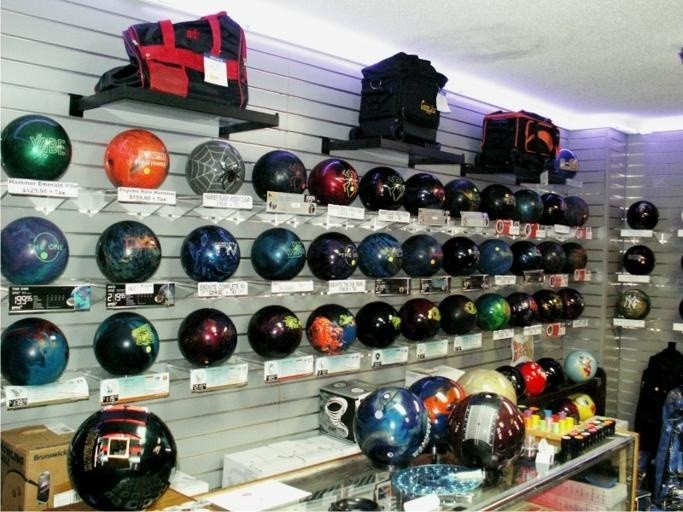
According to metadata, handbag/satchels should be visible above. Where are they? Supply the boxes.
[94,9,249,109]
[475,109,560,174]
[359,50,448,146]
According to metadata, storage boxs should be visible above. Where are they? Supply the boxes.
[1,419,83,510]
